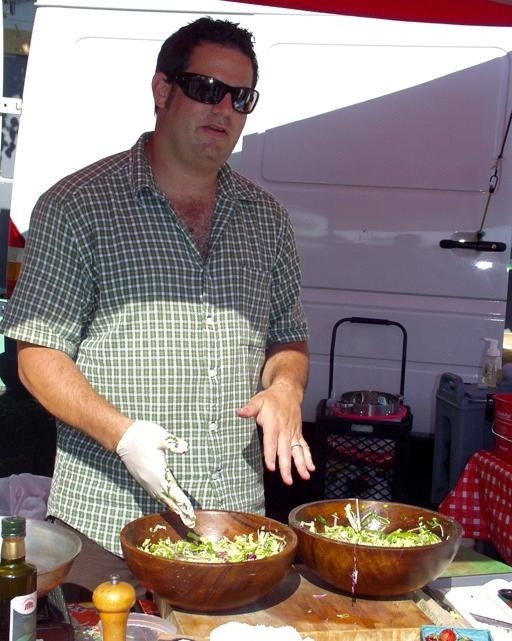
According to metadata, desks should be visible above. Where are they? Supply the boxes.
[437,448,512,565]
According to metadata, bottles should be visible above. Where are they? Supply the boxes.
[0,517,37,640]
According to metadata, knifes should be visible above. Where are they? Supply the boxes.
[469,612,511,629]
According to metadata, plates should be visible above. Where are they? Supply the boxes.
[95,611,178,640]
[426,571,512,619]
[420,624,494,640]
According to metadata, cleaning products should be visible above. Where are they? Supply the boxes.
[478,337,503,389]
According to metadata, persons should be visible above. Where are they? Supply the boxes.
[0,19,316,605]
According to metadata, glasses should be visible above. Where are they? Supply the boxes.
[174,70,262,114]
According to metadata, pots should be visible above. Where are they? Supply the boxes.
[337,389,405,417]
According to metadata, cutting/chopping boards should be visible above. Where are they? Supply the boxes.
[161,564,438,641]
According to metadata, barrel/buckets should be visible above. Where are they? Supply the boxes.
[491,393,512,462]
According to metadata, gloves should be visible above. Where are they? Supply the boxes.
[118,419,196,534]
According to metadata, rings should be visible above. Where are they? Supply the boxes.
[291,444,303,449]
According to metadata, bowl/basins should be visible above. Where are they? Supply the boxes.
[120,509,296,615]
[288,497,464,600]
[0,516,84,600]
